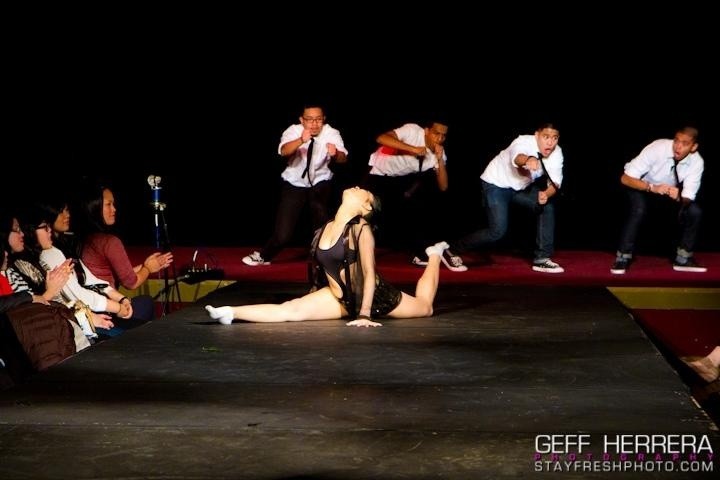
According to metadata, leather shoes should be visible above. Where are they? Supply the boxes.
[412,256,428,267]
[678,354,720,383]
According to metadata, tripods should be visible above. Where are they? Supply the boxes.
[150,189,181,318]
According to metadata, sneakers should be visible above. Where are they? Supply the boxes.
[241,250,272,266]
[532,259,565,274]
[673,255,708,273]
[610,261,628,274]
[441,250,468,273]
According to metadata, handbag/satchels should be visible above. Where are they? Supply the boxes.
[111,295,153,329]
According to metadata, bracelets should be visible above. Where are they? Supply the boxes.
[647,182,655,193]
[527,154,537,160]
[300,135,306,144]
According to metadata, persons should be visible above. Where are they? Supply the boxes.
[241,103,352,269]
[0,185,175,371]
[367,117,451,267]
[438,115,566,274]
[202,185,452,326]
[609,125,709,275]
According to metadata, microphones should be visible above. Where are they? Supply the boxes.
[146,175,161,188]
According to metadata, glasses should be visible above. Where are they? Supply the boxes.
[35,223,50,232]
[11,226,22,234]
